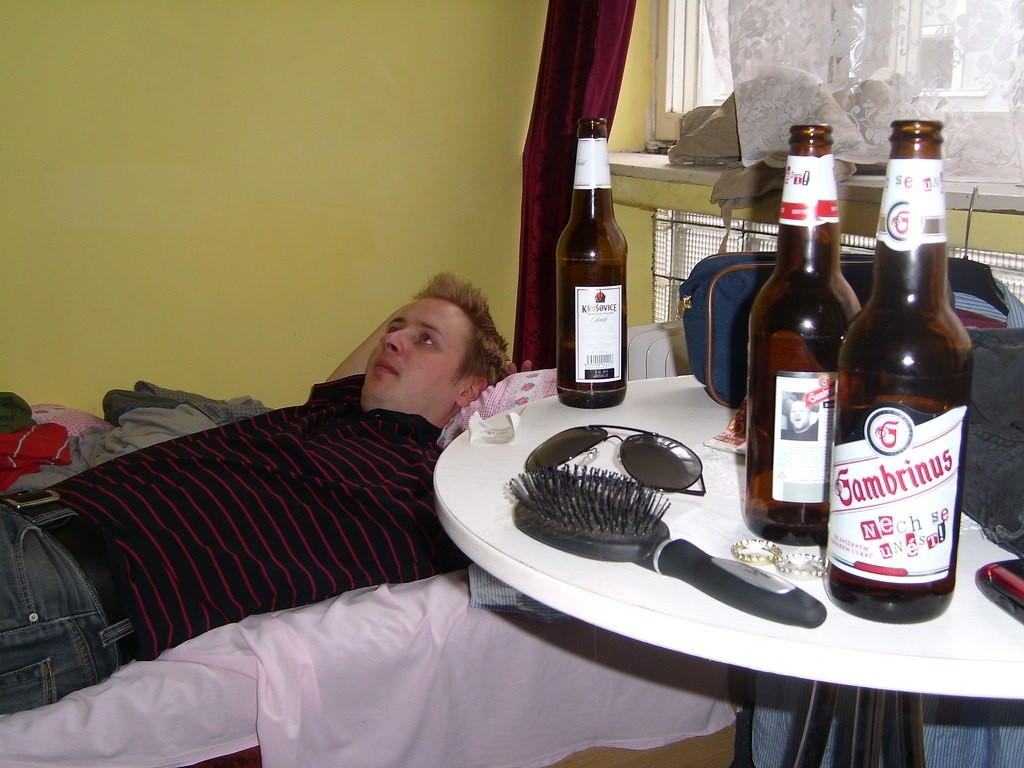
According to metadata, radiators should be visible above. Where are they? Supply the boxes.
[626,319,695,381]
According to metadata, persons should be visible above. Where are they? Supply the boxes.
[0,269,534,716]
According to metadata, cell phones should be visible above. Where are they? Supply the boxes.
[975,559,1024,624]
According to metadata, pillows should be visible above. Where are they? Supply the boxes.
[434,368,558,449]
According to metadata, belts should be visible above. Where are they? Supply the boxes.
[0,488,138,665]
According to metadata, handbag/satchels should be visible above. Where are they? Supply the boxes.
[679,251,1024,408]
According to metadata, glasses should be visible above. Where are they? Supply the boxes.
[526,425,707,496]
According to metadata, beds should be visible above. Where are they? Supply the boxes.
[1,564,738,768]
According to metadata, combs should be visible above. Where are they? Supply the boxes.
[507,462,828,630]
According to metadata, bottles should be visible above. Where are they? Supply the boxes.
[557,117,629,409]
[823,121,974,623]
[742,124,861,546]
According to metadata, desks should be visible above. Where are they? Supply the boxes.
[433,374,1024,767]
